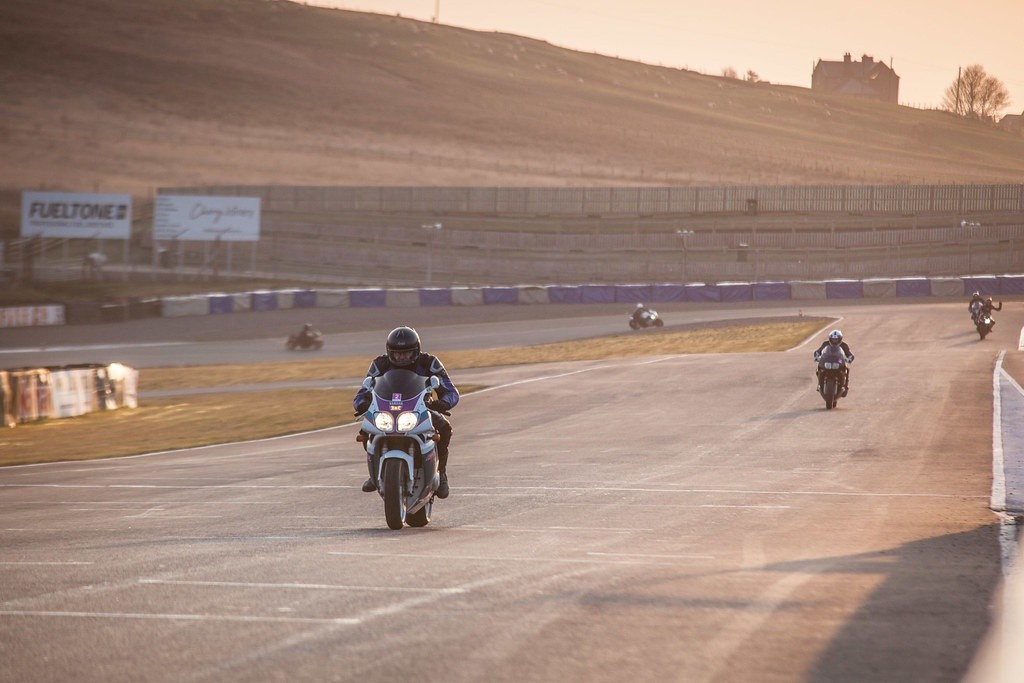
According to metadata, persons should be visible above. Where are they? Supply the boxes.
[633,303,643,319]
[301,323,312,336]
[814,330,854,392]
[353,325,460,499]
[982,298,1002,332]
[968,292,985,319]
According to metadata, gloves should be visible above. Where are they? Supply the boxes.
[999,301,1002,306]
[357,397,370,415]
[431,400,450,414]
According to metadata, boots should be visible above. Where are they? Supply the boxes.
[843,368,849,397]
[362,452,377,492]
[816,371,821,391]
[436,449,449,499]
[989,321,995,332]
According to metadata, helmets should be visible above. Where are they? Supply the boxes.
[386,325,421,368]
[986,297,993,303]
[829,330,843,348]
[972,290,980,297]
[636,303,642,308]
[303,320,313,330]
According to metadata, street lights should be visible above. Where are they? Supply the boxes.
[676,227,695,286]
[421,224,443,288]
[960,219,980,279]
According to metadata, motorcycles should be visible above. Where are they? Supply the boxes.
[353,367,452,530]
[287,329,325,352]
[815,344,853,410]
[975,310,994,341]
[629,309,664,331]
[971,301,984,326]
